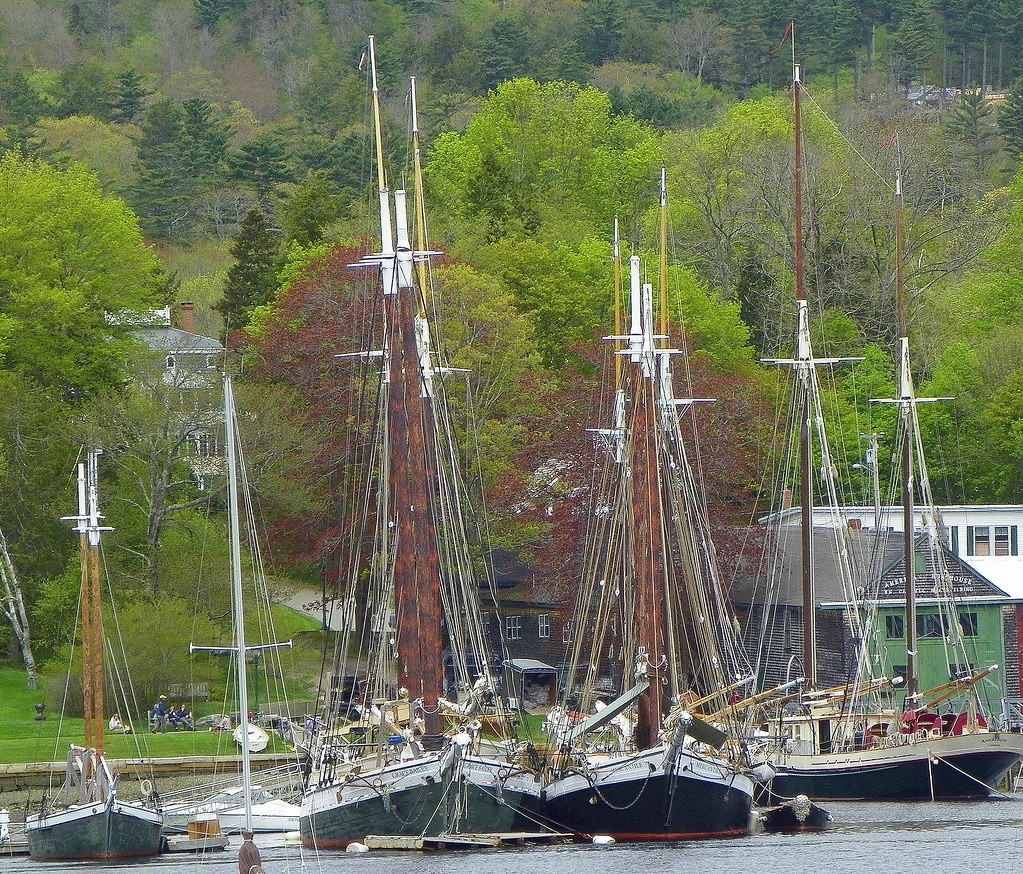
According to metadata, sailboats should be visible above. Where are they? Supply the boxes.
[109,26,1022,855]
[23,444,167,867]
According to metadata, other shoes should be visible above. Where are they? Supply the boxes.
[152,730,156,734]
[194,728,198,731]
[175,729,178,731]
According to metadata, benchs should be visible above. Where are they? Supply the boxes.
[148,709,193,733]
[230,712,265,728]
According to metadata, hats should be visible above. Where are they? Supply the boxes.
[159,695,167,698]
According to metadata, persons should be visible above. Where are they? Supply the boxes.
[108,714,122,730]
[151,695,168,734]
[247,710,254,722]
[210,714,231,731]
[166,704,194,731]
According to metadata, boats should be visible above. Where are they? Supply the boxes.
[232,722,270,754]
